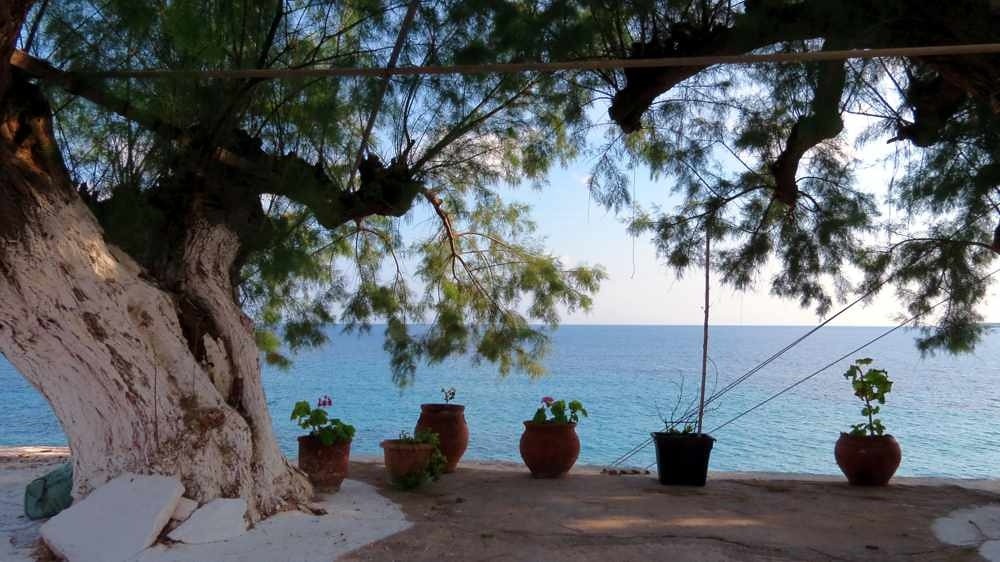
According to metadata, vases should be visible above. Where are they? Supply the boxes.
[519,420,581,477]
[294,433,351,494]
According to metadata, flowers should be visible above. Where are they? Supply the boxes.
[291,394,356,445]
[534,395,588,423]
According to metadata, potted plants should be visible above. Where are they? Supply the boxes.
[649,355,718,484]
[833,356,902,484]
[377,421,443,496]
[413,384,469,470]
